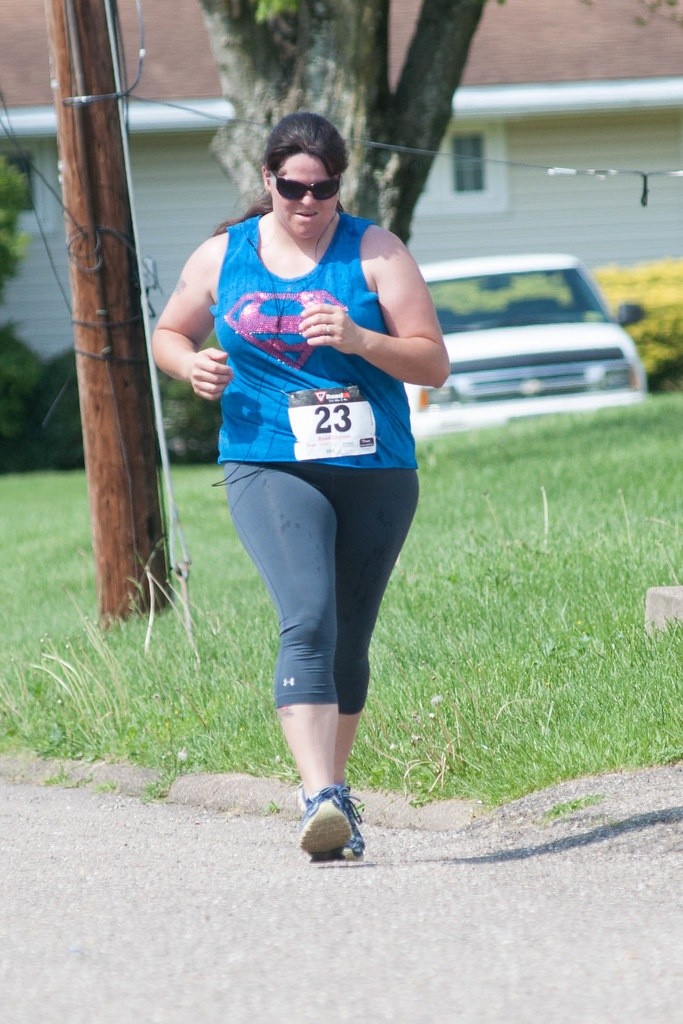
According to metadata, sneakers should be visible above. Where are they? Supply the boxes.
[299,783,365,862]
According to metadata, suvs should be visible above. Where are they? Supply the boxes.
[403,254,649,440]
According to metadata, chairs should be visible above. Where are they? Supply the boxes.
[507,298,559,315]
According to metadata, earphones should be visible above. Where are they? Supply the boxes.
[266,173,271,185]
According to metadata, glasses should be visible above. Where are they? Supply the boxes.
[270,169,341,200]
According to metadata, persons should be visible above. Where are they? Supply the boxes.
[149,111,452,865]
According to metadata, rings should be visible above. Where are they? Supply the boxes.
[326,325,331,334]
